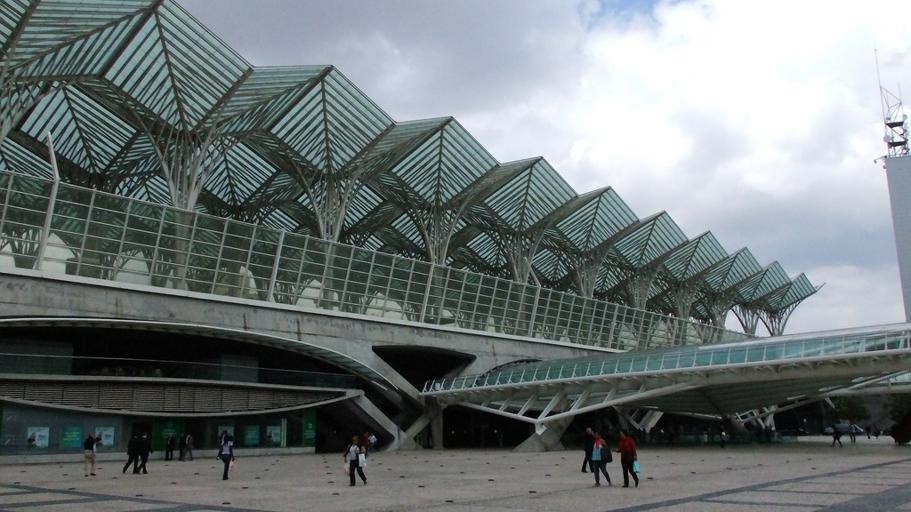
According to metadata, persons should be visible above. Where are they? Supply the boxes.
[220,433,236,479]
[137,432,154,474]
[368,431,379,452]
[83,431,104,478]
[179,430,187,461]
[122,431,140,474]
[824,423,861,448]
[186,431,195,460]
[614,430,640,487]
[342,435,369,487]
[590,430,613,487]
[26,431,38,450]
[165,431,176,461]
[582,427,594,473]
[363,430,371,458]
[706,427,726,448]
[217,429,230,458]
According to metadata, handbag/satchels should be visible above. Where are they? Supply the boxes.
[633,461,640,473]
[600,446,613,463]
[358,453,366,467]
[344,461,350,475]
